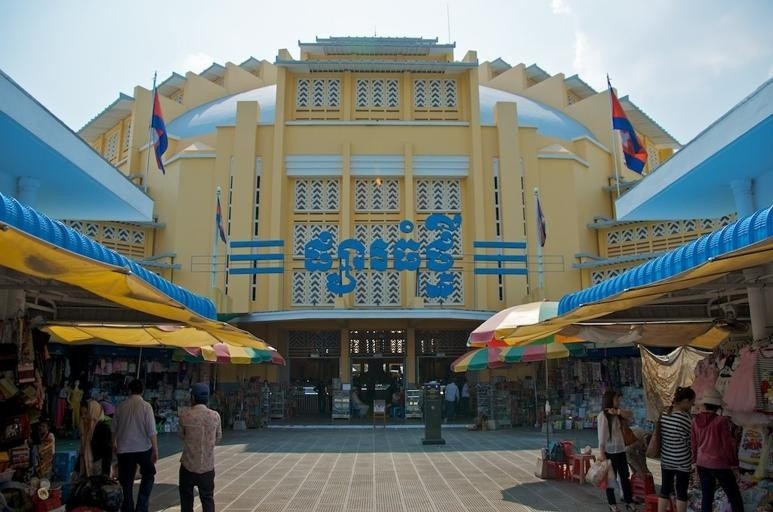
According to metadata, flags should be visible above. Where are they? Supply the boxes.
[534,197,547,248]
[152,89,167,177]
[608,81,647,175]
[216,199,227,246]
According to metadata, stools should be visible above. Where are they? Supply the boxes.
[53,450,77,482]
[570,453,596,484]
[628,471,656,501]
[644,494,674,511]
[389,406,403,417]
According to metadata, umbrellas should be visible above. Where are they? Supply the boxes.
[466,298,560,448]
[449,344,589,430]
[171,341,287,391]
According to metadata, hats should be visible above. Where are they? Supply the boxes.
[189,382,209,398]
[698,387,728,409]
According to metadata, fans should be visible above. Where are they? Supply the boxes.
[711,301,749,337]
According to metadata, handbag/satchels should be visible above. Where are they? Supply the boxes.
[619,414,639,447]
[645,405,669,459]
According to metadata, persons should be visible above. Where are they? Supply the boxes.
[72,398,114,482]
[387,383,403,420]
[111,379,158,512]
[352,386,370,417]
[442,377,459,422]
[314,382,327,414]
[691,389,744,512]
[659,386,695,512]
[179,382,222,512]
[597,391,635,512]
[462,381,471,418]
[37,421,55,475]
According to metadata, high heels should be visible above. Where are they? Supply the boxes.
[608,503,620,512]
[623,502,640,512]
